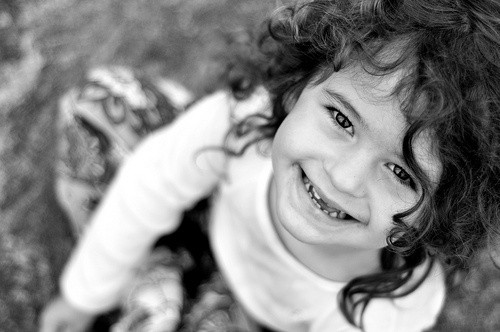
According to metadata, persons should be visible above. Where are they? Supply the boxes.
[32,0,500,332]
